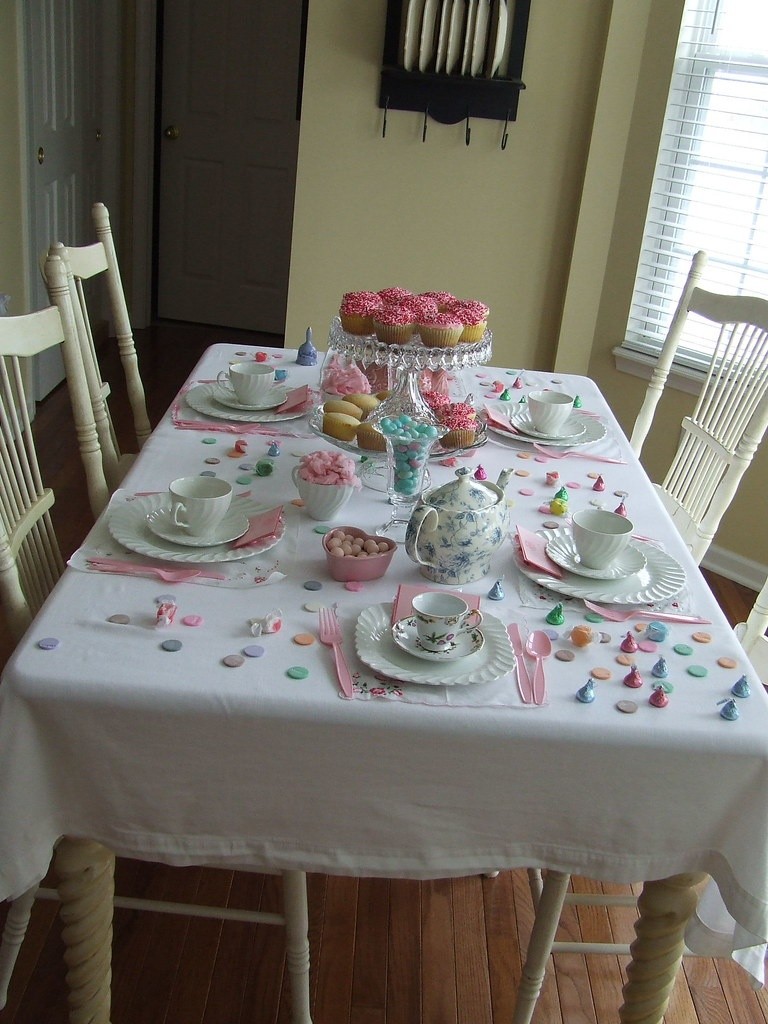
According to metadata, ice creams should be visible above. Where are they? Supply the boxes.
[298,450,360,487]
[321,364,371,395]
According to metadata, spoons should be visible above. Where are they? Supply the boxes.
[565,518,648,540]
[175,424,260,433]
[526,630,551,705]
[89,564,201,582]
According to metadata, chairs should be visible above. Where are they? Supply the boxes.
[0,200,768,1024]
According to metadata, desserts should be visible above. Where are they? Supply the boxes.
[322,390,476,452]
[338,286,489,348]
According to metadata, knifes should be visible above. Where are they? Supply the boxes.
[87,556,225,580]
[506,622,533,703]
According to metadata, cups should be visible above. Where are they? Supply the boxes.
[529,392,574,433]
[291,466,354,521]
[412,591,482,651]
[217,363,275,404]
[573,509,633,569]
[169,476,233,536]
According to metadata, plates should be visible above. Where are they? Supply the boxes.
[483,403,607,445]
[511,415,586,438]
[214,386,287,410]
[545,536,647,579]
[109,494,285,563]
[355,606,516,685]
[514,527,685,604]
[186,382,315,422]
[148,507,251,546]
[393,616,485,661]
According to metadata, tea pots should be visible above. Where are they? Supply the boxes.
[406,462,515,585]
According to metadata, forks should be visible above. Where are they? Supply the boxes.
[135,490,252,497]
[533,443,627,463]
[320,608,353,698]
[584,599,712,624]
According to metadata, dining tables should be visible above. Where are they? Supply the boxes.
[0,341,768,1024]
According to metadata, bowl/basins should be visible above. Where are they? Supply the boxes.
[322,527,398,582]
